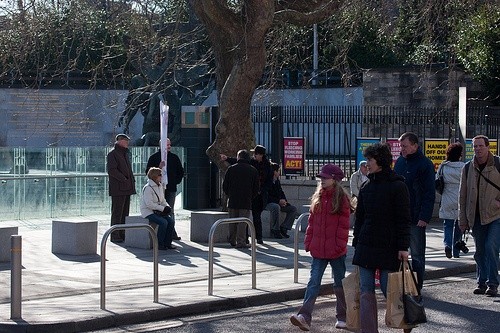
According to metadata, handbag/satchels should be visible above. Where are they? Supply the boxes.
[402,258,428,325]
[384,258,428,328]
[341,265,361,328]
[154,210,164,216]
[434,164,443,195]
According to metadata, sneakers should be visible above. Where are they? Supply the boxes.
[486,286,497,294]
[290,314,310,331]
[473,285,486,293]
[335,320,347,328]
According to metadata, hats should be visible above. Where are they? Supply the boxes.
[117,134,130,140]
[316,164,345,182]
[250,145,266,154]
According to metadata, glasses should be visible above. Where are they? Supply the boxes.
[158,174,161,176]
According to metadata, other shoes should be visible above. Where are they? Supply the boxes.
[174,236,180,240]
[280,227,289,238]
[168,245,175,249]
[237,244,251,248]
[445,246,452,258]
[271,228,282,239]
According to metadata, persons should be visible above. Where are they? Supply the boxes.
[145,138,184,240]
[351,143,411,333]
[438,142,469,259]
[289,164,350,329]
[220,145,297,248]
[393,132,436,302]
[458,135,500,294]
[105,134,137,242]
[140,167,177,250]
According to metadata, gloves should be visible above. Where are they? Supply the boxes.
[164,206,171,212]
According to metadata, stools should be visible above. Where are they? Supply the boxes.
[191,211,229,243]
[52,220,98,255]
[249,210,286,236]
[125,215,159,248]
[0,222,19,263]
[301,205,310,231]
